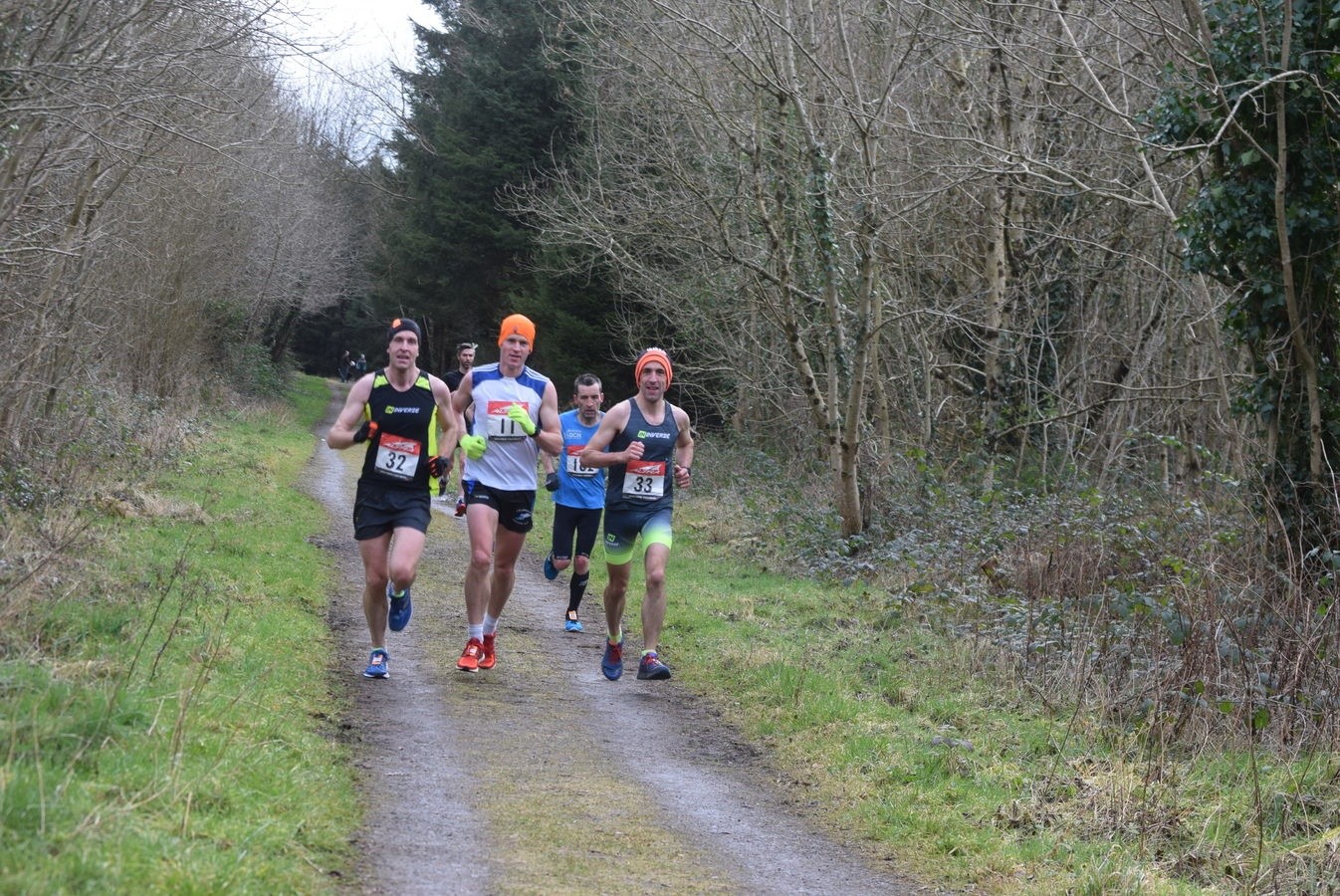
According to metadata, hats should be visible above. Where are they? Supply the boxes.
[635,347,672,392]
[386,318,422,349]
[499,314,536,352]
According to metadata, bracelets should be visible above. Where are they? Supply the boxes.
[528,426,540,437]
[680,467,691,476]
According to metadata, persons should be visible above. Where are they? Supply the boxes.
[453,313,564,673]
[326,318,458,680]
[579,347,694,680]
[356,353,366,381]
[540,373,608,632]
[436,340,476,517]
[339,350,351,382]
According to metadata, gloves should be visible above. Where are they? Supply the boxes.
[354,420,379,443]
[458,435,486,461]
[427,455,450,479]
[544,473,559,492]
[508,402,539,436]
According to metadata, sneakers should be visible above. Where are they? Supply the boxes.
[636,652,672,680]
[455,498,466,517]
[478,632,497,671]
[439,479,447,496]
[544,550,560,580]
[601,634,624,680]
[387,580,412,632]
[565,610,584,633]
[456,638,485,673]
[364,646,389,678]
